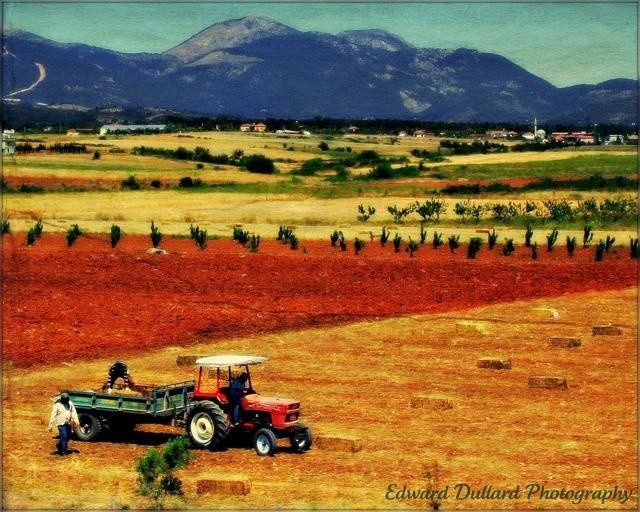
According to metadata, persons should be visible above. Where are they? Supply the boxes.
[47,392,80,455]
[229,371,248,425]
[104,360,138,395]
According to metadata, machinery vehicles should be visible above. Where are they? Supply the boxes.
[50,355,313,457]
